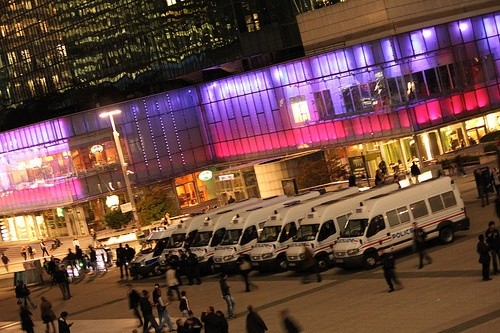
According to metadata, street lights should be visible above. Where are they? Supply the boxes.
[98,109,146,246]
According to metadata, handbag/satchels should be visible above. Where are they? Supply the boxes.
[240,261,251,270]
[168,279,176,286]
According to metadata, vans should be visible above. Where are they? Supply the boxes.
[249,182,361,274]
[184,192,289,278]
[285,180,402,272]
[211,189,321,276]
[332,171,472,270]
[128,197,261,278]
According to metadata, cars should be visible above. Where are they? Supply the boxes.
[472,130,500,153]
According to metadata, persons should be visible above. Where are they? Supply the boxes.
[411,221,433,269]
[164,266,180,301]
[14,279,38,333]
[165,249,202,285]
[379,248,404,293]
[219,275,235,317]
[164,212,173,226]
[476,220,500,281]
[50,238,61,251]
[39,296,57,333]
[40,238,50,257]
[246,304,269,333]
[115,242,136,279]
[20,244,36,260]
[473,165,500,218]
[279,308,303,333]
[58,312,74,333]
[342,160,421,186]
[300,243,322,283]
[237,252,257,293]
[124,282,229,333]
[1,253,11,272]
[40,243,98,300]
[90,228,98,247]
